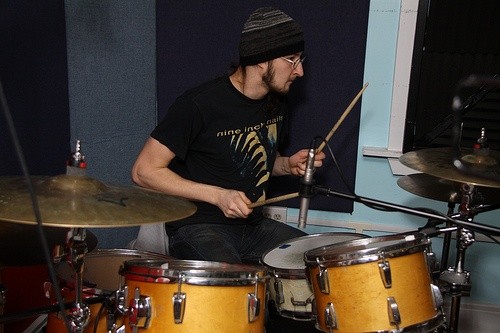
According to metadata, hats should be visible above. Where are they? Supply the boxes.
[239,6,305,66]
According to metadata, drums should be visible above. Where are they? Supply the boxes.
[45,248,177,333]
[118,257,269,333]
[303,232,447,333]
[257,231,372,323]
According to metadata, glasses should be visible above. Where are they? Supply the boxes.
[281,57,305,69]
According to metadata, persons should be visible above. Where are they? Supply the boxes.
[131,6,326,333]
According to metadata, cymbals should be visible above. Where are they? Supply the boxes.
[396,172,500,209]
[0,172,198,230]
[398,145,500,189]
